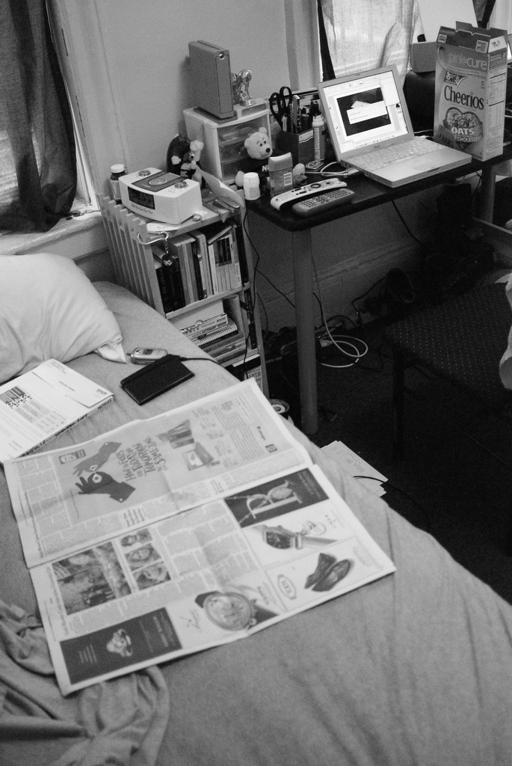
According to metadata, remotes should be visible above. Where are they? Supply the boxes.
[269,176,348,212]
[292,186,356,216]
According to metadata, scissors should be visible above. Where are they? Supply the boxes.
[269,86,293,132]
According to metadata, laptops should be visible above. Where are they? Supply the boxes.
[316,63,474,189]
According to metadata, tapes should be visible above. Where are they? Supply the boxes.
[269,398,291,419]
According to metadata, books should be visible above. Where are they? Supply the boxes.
[1,358,116,469]
[150,217,265,394]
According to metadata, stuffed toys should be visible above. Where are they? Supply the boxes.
[233,126,307,198]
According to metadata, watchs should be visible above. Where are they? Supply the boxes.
[250,522,336,552]
[194,589,277,633]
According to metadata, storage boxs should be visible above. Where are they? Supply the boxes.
[434,23,508,162]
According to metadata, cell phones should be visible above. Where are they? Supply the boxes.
[127,344,168,364]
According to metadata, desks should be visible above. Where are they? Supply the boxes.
[239,148,512,435]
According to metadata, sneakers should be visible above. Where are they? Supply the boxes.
[304,552,350,592]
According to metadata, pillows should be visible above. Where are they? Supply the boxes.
[0,254,123,382]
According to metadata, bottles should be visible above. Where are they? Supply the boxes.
[110,163,128,205]
[242,172,261,201]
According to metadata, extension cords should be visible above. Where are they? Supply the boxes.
[341,300,393,331]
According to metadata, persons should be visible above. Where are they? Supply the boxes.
[78,568,116,608]
[135,564,171,590]
[121,528,154,549]
[126,546,160,571]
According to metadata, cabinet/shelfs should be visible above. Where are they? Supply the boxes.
[96,187,270,411]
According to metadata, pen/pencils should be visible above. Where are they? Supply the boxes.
[301,94,320,129]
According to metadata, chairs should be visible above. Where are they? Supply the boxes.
[385,282,512,460]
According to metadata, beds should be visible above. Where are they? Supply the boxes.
[0,282,512,766]
